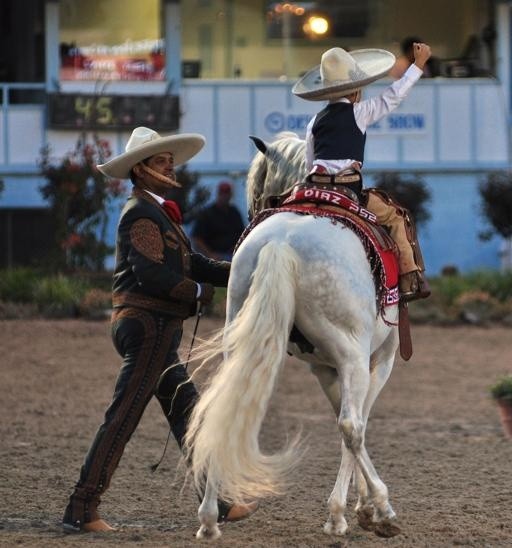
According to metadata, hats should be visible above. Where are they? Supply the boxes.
[287,45,397,102]
[93,122,207,180]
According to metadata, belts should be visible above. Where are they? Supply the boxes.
[308,170,363,186]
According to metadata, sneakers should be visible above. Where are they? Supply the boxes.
[400,268,435,302]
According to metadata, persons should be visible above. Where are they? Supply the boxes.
[191,174,246,319]
[60,126,259,535]
[386,36,423,79]
[293,37,435,304]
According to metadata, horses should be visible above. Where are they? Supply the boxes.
[156,131,400,542]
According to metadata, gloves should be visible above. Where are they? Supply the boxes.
[198,283,215,308]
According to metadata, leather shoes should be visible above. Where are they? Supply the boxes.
[202,497,261,522]
[60,508,123,536]
